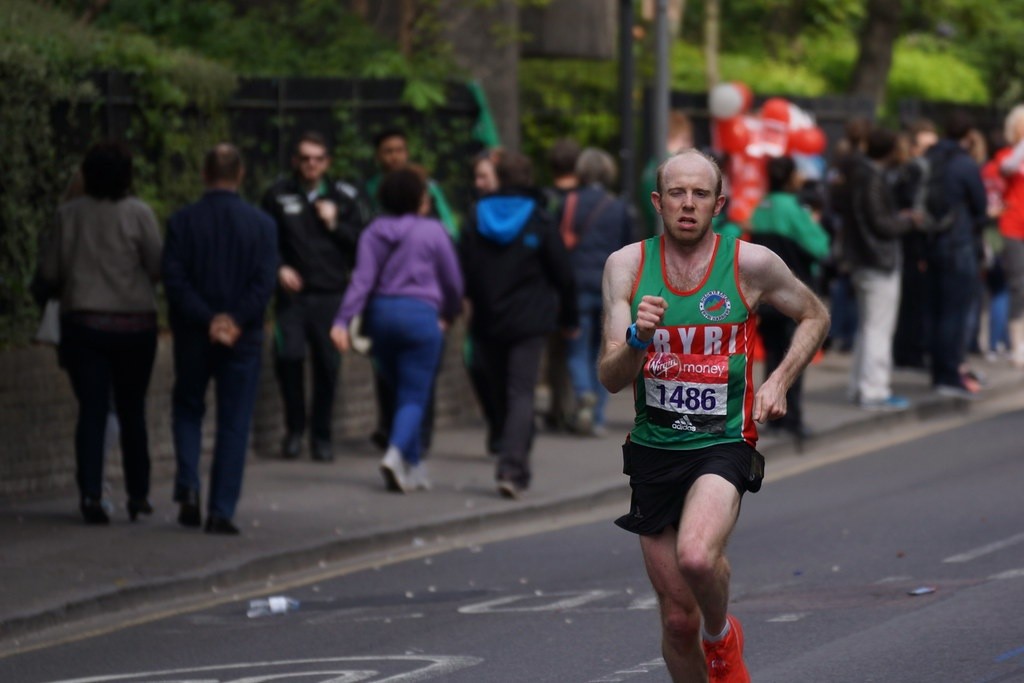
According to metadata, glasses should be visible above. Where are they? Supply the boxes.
[297,154,327,164]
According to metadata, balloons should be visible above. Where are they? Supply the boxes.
[706,81,827,225]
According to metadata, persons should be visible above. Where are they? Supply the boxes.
[641,110,739,238]
[803,109,1024,410]
[473,154,501,398]
[168,142,281,534]
[560,148,636,437]
[598,147,831,683]
[26,140,161,523]
[363,130,456,460]
[701,149,744,239]
[542,145,579,432]
[459,151,569,496]
[332,169,467,491]
[262,128,362,463]
[748,157,827,438]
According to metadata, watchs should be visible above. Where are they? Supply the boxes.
[626,326,652,350]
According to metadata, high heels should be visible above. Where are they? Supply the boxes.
[83,506,111,524]
[126,500,154,522]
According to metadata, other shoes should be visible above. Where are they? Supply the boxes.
[937,373,987,402]
[498,480,520,499]
[381,455,408,493]
[283,432,301,458]
[178,490,201,527]
[204,516,240,535]
[862,394,911,413]
[311,438,333,460]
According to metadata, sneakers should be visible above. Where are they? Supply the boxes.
[701,615,750,683]
